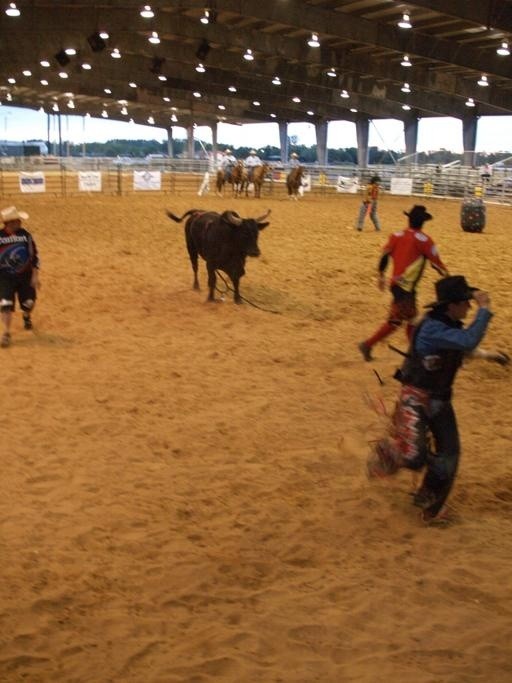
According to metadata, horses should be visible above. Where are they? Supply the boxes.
[216,159,248,199]
[285,165,306,202]
[241,161,272,199]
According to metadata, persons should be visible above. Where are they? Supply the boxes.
[0,203,40,347]
[356,175,384,233]
[365,275,510,525]
[219,147,237,181]
[287,151,303,175]
[357,203,453,361]
[480,162,493,182]
[244,149,263,180]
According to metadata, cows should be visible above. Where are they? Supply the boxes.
[163,207,271,307]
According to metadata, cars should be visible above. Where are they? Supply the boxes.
[115,151,289,171]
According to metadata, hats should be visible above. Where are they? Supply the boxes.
[422,275,480,308]
[0,206,29,222]
[225,149,300,157]
[403,205,432,220]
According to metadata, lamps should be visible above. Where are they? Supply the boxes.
[87,33,106,52]
[196,39,211,60]
[209,10,218,24]
[242,48,254,61]
[150,54,166,75]
[307,14,510,99]
[53,47,69,67]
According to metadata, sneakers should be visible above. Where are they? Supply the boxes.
[357,342,373,361]
[358,228,362,231]
[414,513,447,527]
[0,333,11,347]
[375,229,380,231]
[22,316,33,329]
